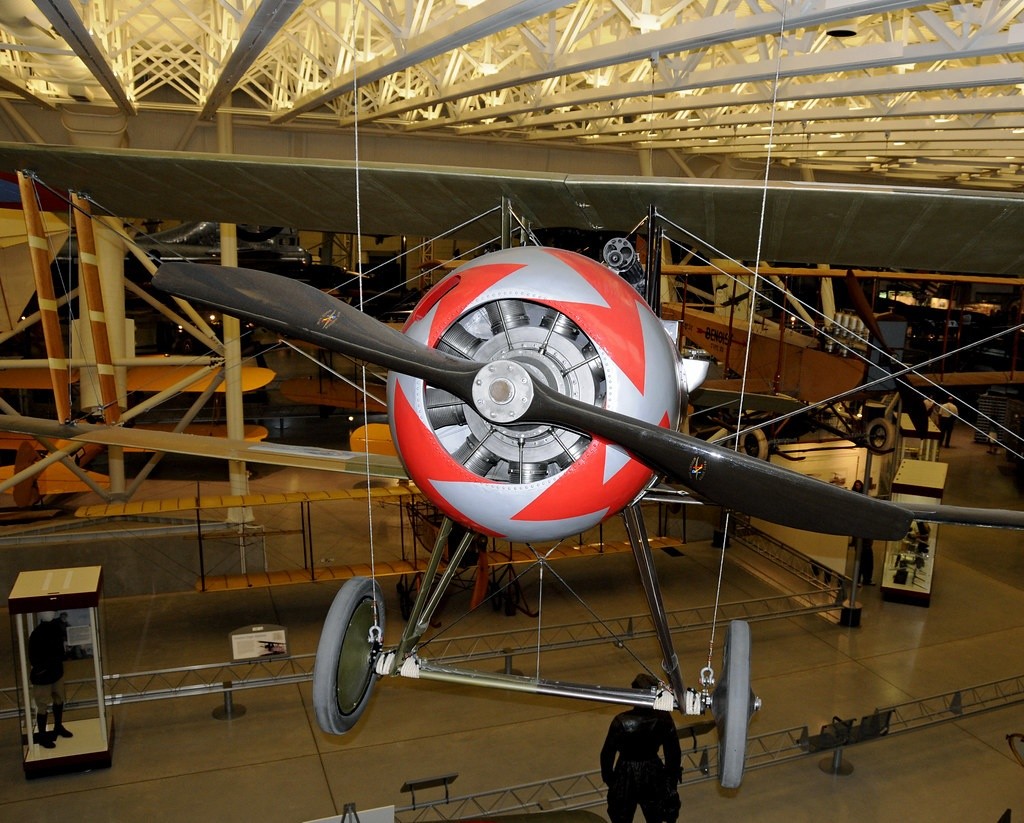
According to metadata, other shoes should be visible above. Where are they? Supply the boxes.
[939,442,951,448]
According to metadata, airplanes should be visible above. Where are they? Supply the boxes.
[151,187,1022,788]
[630,207,1022,469]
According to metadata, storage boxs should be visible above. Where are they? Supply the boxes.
[880,458,949,609]
[6,564,118,781]
[892,412,941,479]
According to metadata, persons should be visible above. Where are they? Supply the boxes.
[28,610,73,748]
[937,397,959,449]
[923,393,936,417]
[600,672,684,823]
[852,479,863,493]
[848,537,874,586]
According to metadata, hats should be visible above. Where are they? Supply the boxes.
[631,674,659,690]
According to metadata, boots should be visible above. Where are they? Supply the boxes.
[36,702,73,748]
